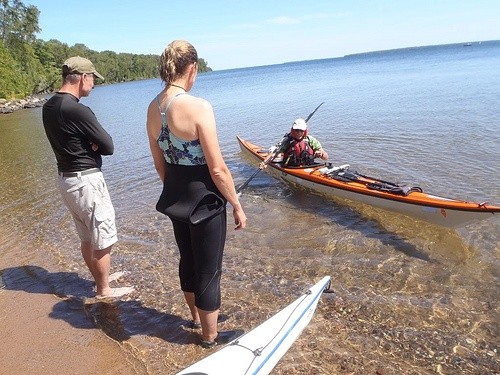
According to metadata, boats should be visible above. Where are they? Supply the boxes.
[236,134,500,230]
[171,275,333,375]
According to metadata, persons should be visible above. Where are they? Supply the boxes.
[42,56,135,299]
[259,118,329,170]
[146,39,247,349]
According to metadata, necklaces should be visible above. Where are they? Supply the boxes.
[166,83,187,92]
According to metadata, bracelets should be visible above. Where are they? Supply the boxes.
[321,153,324,158]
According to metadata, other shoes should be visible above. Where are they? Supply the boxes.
[191,314,245,349]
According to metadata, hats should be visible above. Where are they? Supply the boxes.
[291,119,307,131]
[60,56,105,82]
